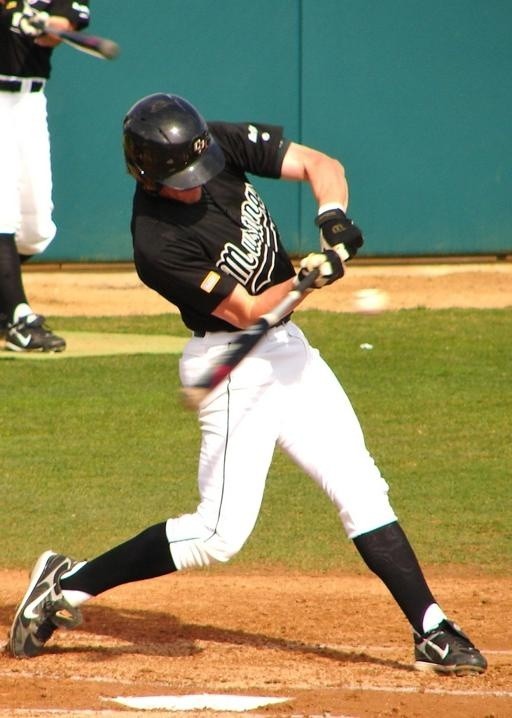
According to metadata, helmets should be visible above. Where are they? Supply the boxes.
[122,92,227,192]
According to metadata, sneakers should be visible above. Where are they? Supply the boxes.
[414,619,488,674]
[6,312,66,353]
[10,551,82,659]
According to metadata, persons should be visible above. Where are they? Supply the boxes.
[9,92,486,672]
[0,0,95,356]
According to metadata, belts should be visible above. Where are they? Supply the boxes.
[0,79,44,93]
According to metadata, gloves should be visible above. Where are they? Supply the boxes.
[297,207,364,289]
[0,0,50,38]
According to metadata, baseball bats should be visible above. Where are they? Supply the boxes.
[37,20,117,61]
[178,268,319,412]
